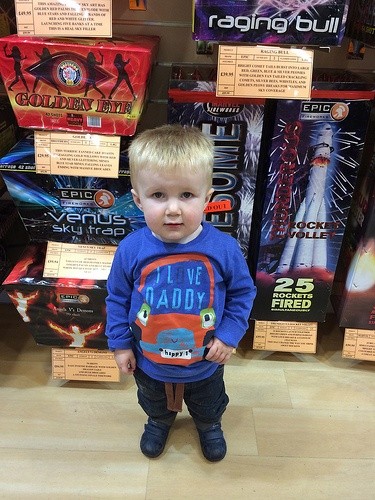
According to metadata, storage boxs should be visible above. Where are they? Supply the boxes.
[0,0,374,349]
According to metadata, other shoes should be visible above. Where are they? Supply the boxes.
[140,414,173,458]
[193,418,227,462]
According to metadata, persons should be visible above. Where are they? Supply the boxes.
[105,122,257,463]
